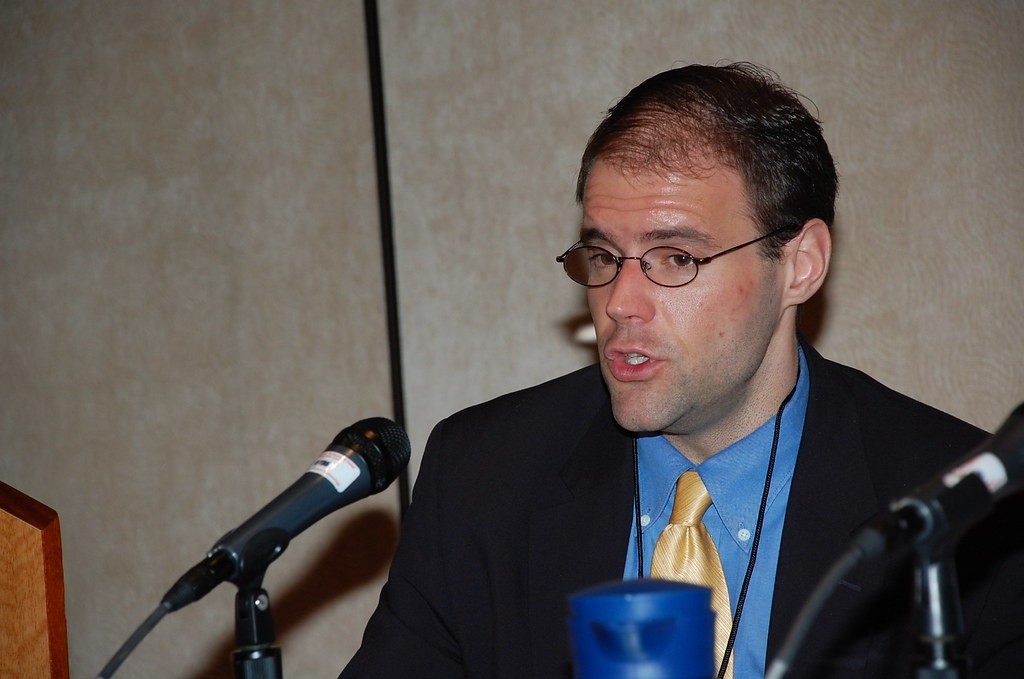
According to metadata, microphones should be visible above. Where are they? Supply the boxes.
[856,402,1024,557]
[162,417,411,613]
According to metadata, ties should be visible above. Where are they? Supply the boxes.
[649,466,734,679]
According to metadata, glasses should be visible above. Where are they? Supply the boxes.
[555,219,814,289]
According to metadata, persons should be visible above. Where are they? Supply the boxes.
[337,58,1024,679]
